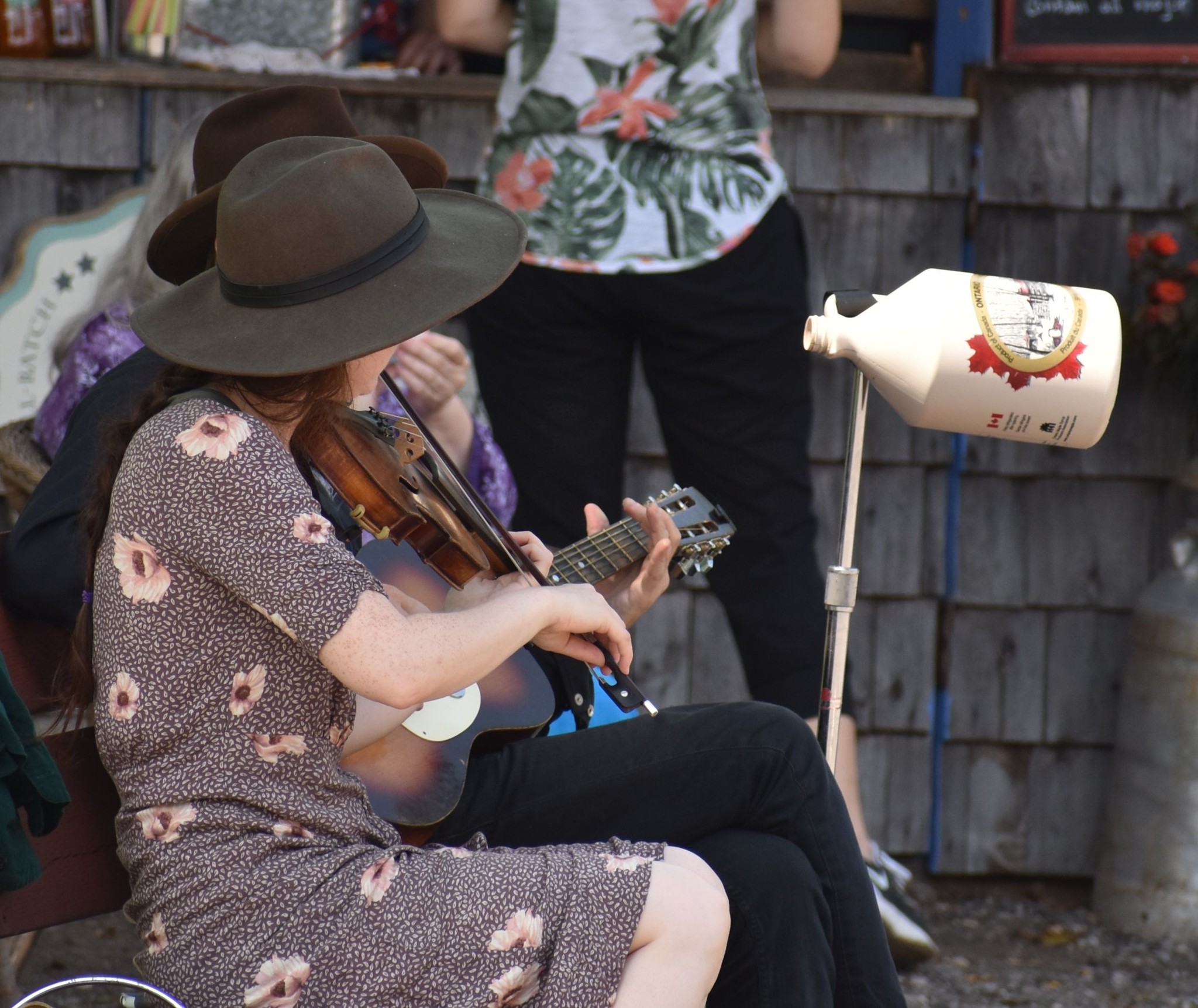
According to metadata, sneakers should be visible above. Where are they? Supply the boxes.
[868,862,937,973]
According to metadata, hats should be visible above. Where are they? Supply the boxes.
[146,84,448,286]
[129,134,528,378]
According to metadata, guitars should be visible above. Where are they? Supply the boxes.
[338,483,738,847]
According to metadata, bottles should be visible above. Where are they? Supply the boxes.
[801,268,1122,450]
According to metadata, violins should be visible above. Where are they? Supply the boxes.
[294,398,525,592]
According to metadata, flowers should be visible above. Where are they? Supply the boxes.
[1120,232,1198,346]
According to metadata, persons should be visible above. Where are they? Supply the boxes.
[432,0,938,972]
[1,83,908,1008]
